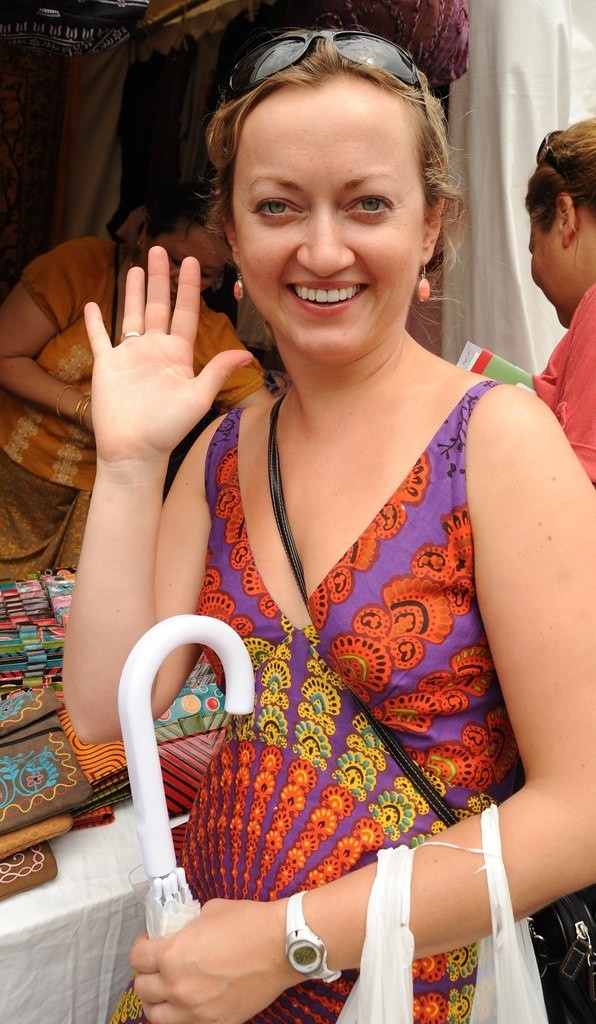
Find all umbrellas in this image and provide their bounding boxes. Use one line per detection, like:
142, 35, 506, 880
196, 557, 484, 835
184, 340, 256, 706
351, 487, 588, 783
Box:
117, 613, 255, 944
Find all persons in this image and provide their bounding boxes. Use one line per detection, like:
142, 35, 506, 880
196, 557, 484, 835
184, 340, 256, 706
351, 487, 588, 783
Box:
0, 180, 273, 582
525, 118, 596, 486
63, 29, 596, 1024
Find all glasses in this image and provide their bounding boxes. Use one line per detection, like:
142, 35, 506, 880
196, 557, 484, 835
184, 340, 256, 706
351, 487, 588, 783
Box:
537, 128, 578, 208
223, 30, 428, 123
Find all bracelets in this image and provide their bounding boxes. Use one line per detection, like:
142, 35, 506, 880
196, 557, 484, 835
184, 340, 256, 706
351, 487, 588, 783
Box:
74, 395, 91, 426
56, 385, 72, 418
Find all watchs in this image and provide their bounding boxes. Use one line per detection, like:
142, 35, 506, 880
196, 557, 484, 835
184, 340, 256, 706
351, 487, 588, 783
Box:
285, 891, 342, 983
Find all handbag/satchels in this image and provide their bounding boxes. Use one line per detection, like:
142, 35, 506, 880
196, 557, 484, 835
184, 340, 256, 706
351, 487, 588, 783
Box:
526, 892, 596, 1024
331, 802, 548, 1024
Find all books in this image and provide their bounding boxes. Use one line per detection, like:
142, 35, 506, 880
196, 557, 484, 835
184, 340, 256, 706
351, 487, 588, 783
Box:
456, 341, 536, 391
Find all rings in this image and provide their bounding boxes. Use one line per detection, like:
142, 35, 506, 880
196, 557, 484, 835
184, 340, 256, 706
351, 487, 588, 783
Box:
121, 331, 143, 341
